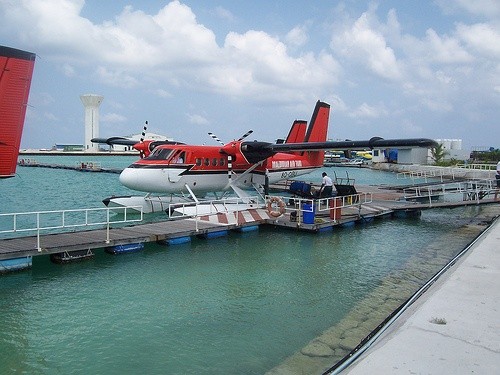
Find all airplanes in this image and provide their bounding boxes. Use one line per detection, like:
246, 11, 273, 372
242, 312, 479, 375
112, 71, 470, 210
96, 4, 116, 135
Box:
90, 100, 441, 218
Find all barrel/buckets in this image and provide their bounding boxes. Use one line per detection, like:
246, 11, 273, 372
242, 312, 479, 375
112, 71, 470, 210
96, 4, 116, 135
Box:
290, 212, 296, 221
303, 204, 315, 223
290, 182, 311, 193
330, 199, 341, 219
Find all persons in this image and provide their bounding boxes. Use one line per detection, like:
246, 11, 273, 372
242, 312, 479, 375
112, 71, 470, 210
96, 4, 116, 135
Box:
320, 172, 338, 205
493, 160, 500, 200
169, 152, 183, 163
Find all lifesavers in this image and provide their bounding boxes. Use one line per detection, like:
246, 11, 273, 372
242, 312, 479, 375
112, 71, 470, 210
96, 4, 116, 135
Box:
267, 197, 286, 217
